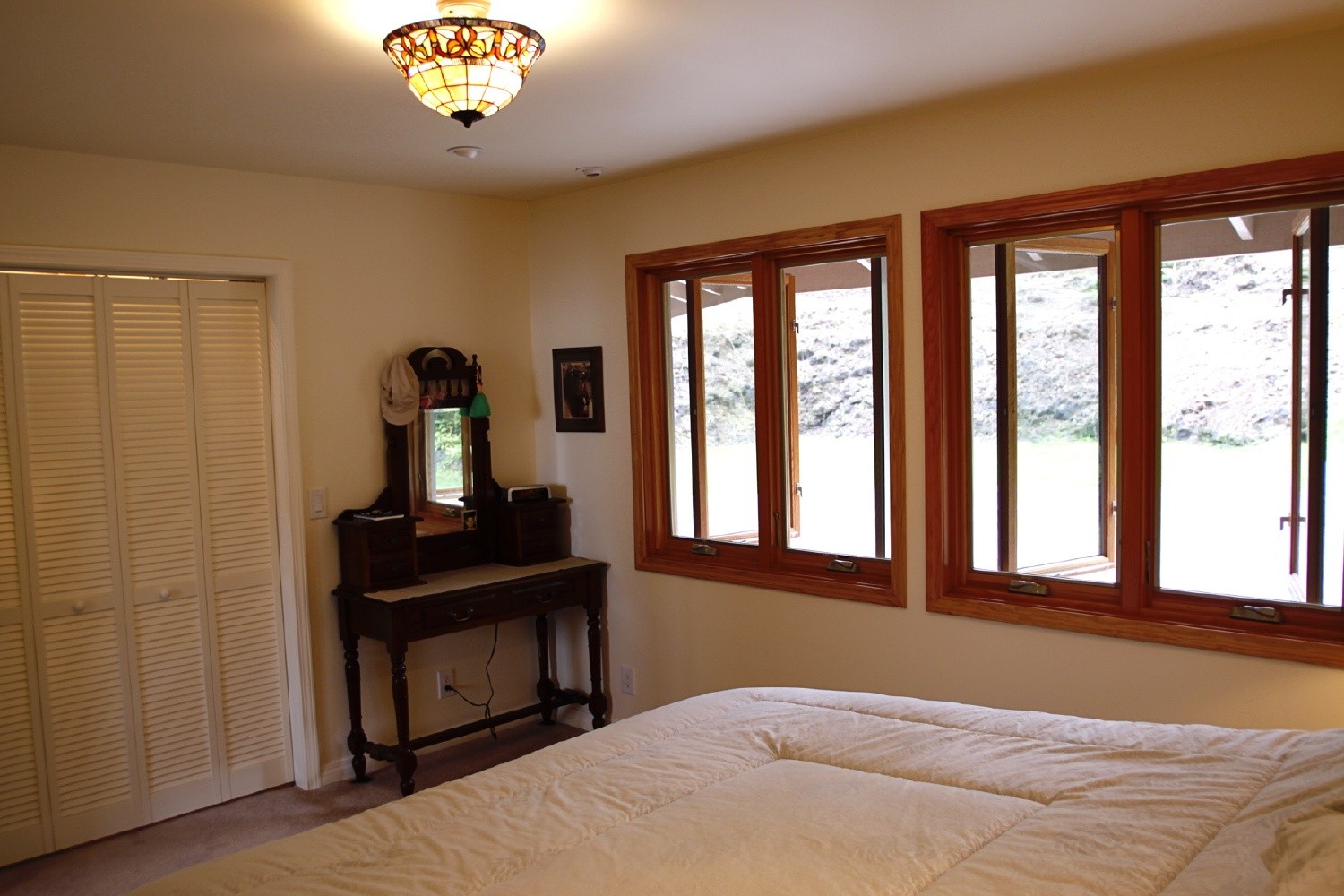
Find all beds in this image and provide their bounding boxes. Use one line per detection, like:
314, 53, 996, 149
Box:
121, 685, 1342, 896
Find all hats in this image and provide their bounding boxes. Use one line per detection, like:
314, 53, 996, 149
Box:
377, 353, 421, 425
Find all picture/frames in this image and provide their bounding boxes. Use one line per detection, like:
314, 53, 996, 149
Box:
552, 345, 605, 432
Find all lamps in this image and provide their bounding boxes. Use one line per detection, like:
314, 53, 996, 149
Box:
468, 366, 491, 418
383, 0, 546, 129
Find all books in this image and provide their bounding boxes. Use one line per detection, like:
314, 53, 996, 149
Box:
353, 512, 405, 521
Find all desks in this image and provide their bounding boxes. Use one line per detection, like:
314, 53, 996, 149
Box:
337, 558, 612, 801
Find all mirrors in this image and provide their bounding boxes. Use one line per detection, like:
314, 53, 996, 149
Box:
381, 345, 493, 541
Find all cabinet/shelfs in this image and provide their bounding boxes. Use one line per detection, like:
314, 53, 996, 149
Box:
333, 510, 423, 593
485, 489, 566, 566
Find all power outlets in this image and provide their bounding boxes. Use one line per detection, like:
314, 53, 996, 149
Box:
436, 669, 455, 699
621, 666, 635, 695
